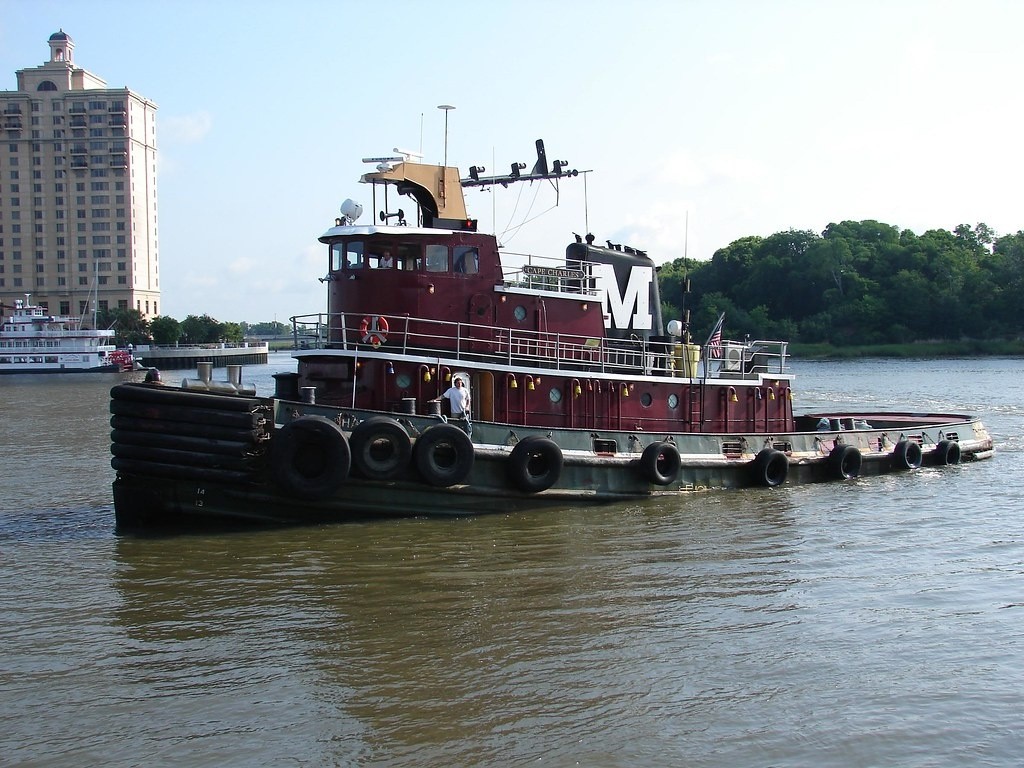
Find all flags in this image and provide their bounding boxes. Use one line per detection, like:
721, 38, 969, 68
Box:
702, 319, 723, 359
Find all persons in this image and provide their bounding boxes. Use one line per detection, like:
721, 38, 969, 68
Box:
427, 378, 470, 418
377, 250, 393, 267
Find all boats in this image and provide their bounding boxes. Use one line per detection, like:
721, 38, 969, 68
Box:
1, 258, 157, 375
102, 349, 134, 373
108, 109, 995, 541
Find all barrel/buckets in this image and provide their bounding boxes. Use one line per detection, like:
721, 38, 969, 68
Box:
677, 344, 700, 377
647, 334, 676, 377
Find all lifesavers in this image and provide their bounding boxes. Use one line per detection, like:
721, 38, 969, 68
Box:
754, 447, 790, 488
411, 421, 475, 488
893, 441, 923, 470
641, 442, 682, 484
935, 440, 962, 466
505, 435, 564, 495
360, 315, 390, 347
272, 414, 352, 496
344, 415, 413, 481
829, 443, 863, 481
